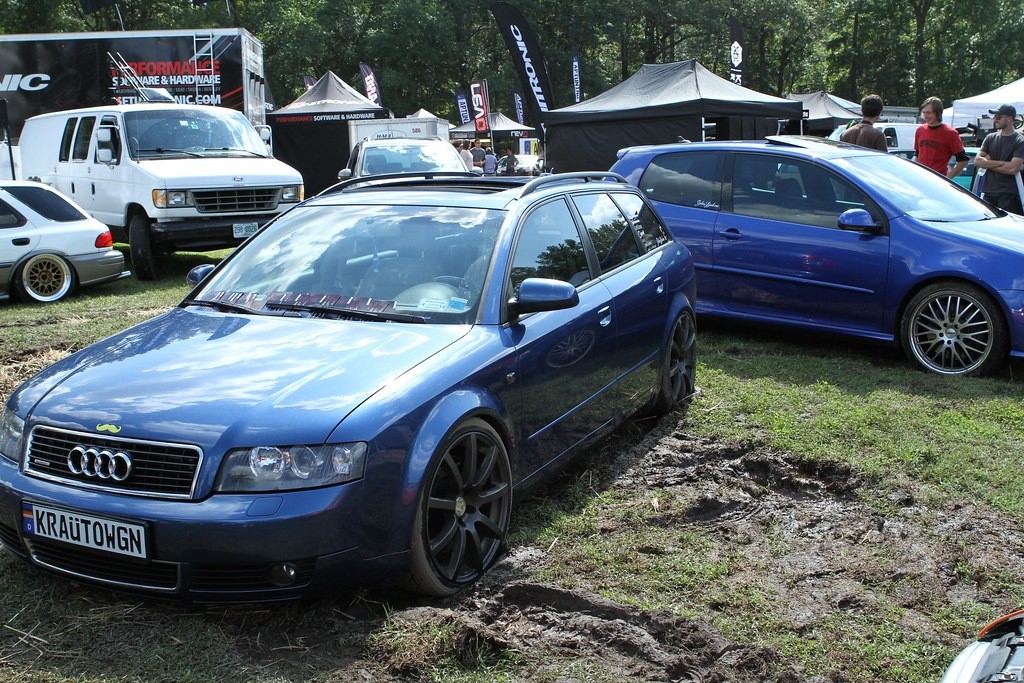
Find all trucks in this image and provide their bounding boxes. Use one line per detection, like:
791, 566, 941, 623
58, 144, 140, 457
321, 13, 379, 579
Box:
348, 118, 450, 164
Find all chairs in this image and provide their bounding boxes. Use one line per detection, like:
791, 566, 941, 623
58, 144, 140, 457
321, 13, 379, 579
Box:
368, 155, 389, 174
354, 216, 444, 302
773, 178, 814, 222
410, 162, 435, 169
461, 216, 550, 314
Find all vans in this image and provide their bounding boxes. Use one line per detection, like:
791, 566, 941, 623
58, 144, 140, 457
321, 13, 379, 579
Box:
825, 123, 923, 150
17, 103, 304, 282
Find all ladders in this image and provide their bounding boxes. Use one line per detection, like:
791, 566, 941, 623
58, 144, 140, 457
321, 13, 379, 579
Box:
193, 31, 215, 106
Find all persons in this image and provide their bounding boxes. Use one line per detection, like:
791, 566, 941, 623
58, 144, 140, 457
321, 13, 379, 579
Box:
973, 103, 1024, 215
910, 96, 971, 180
501, 146, 519, 175
459, 136, 499, 175
840, 93, 888, 153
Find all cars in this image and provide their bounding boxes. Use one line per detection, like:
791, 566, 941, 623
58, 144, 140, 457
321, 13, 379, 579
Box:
0, 180, 132, 303
775, 163, 840, 198
602, 134, 1023, 379
497, 155, 543, 173
887, 147, 981, 193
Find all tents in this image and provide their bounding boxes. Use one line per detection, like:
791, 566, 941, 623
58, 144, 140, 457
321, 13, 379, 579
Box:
942, 77, 1024, 147
265, 69, 390, 201
540, 56, 889, 174
405, 107, 536, 176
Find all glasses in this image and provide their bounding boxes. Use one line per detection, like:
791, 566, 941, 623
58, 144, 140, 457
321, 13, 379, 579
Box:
993, 115, 1009, 120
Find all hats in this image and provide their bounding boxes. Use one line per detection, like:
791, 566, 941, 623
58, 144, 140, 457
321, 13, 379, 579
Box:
988, 105, 1016, 117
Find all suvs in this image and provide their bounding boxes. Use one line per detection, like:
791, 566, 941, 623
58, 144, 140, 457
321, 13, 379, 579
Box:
0, 171, 698, 608
337, 137, 484, 188
